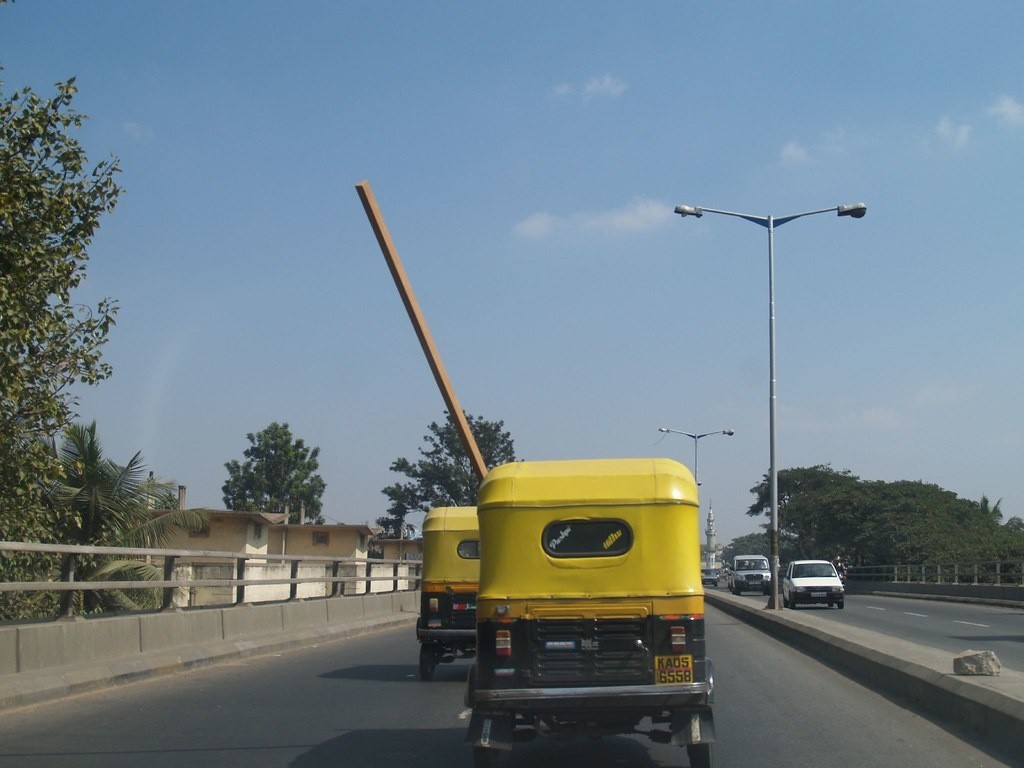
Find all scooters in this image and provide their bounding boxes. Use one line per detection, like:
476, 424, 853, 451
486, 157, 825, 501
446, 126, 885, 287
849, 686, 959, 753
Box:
831, 561, 848, 590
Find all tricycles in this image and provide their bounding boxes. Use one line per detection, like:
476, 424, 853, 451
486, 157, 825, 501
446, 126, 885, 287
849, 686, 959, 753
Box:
415, 506, 479, 676
467, 458, 718, 768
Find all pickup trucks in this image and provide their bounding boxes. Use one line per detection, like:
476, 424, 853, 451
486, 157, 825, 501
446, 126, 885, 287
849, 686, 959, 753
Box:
728, 554, 772, 595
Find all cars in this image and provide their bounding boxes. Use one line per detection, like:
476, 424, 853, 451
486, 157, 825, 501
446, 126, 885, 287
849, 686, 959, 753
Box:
782, 560, 845, 610
701, 568, 720, 587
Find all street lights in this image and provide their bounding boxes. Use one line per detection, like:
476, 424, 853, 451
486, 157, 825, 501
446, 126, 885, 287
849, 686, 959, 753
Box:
659, 427, 735, 486
673, 201, 867, 611
